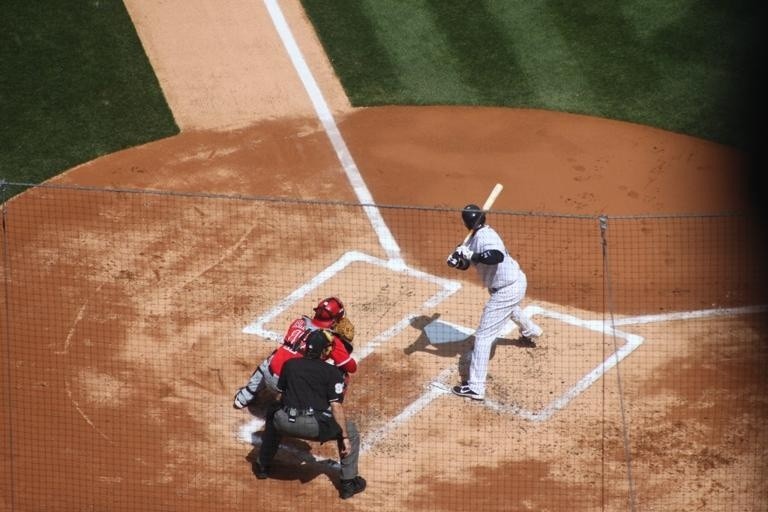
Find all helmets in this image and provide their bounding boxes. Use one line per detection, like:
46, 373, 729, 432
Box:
313, 298, 345, 326
461, 204, 485, 228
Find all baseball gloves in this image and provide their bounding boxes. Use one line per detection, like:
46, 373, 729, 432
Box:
332, 318, 353, 341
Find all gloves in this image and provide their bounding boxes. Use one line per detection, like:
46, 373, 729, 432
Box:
446, 245, 472, 267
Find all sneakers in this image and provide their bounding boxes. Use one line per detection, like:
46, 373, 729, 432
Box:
234, 388, 247, 410
523, 327, 545, 337
452, 384, 485, 400
343, 475, 366, 496
251, 451, 272, 478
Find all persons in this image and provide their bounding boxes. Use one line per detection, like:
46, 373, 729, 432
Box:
234, 297, 357, 409
253, 329, 367, 500
447, 205, 543, 400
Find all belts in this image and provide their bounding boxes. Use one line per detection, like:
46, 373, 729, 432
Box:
282, 406, 305, 416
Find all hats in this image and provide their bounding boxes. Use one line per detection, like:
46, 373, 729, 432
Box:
303, 328, 334, 360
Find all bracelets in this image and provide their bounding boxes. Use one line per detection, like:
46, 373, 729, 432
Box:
342, 436, 350, 439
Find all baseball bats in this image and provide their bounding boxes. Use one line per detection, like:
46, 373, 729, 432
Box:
447, 184, 502, 266
